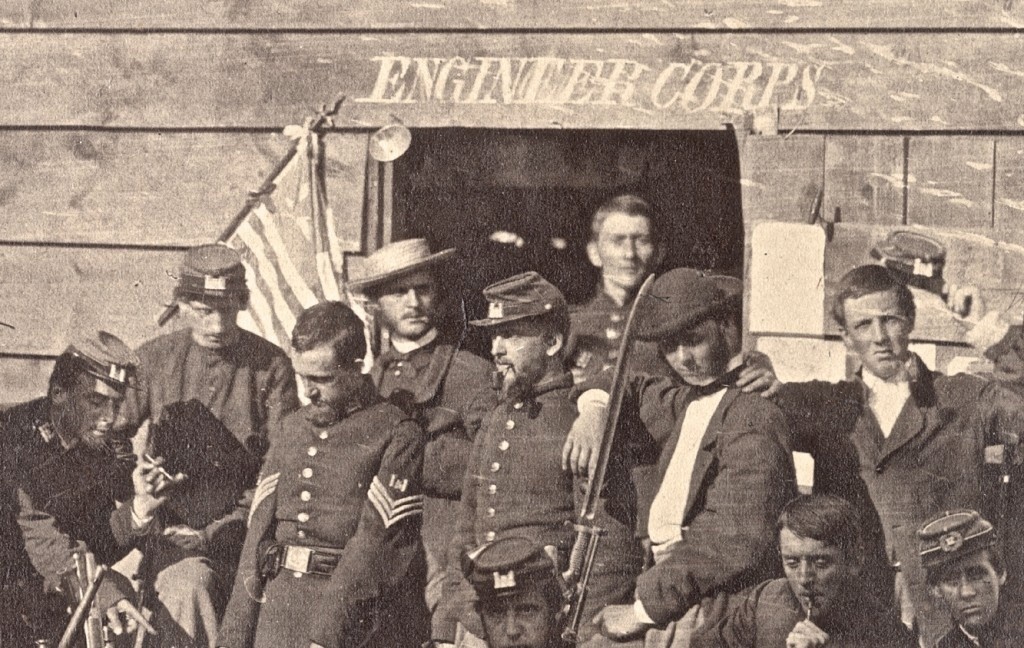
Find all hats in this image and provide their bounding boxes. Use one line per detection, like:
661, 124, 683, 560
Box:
176, 243, 249, 298
870, 231, 952, 299
346, 237, 457, 291
468, 272, 567, 325
916, 509, 1000, 568
469, 535, 556, 601
67, 331, 141, 391
634, 268, 745, 341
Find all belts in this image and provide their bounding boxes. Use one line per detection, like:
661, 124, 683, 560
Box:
280, 545, 338, 575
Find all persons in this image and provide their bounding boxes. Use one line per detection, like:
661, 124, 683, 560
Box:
0, 192, 1024, 648
